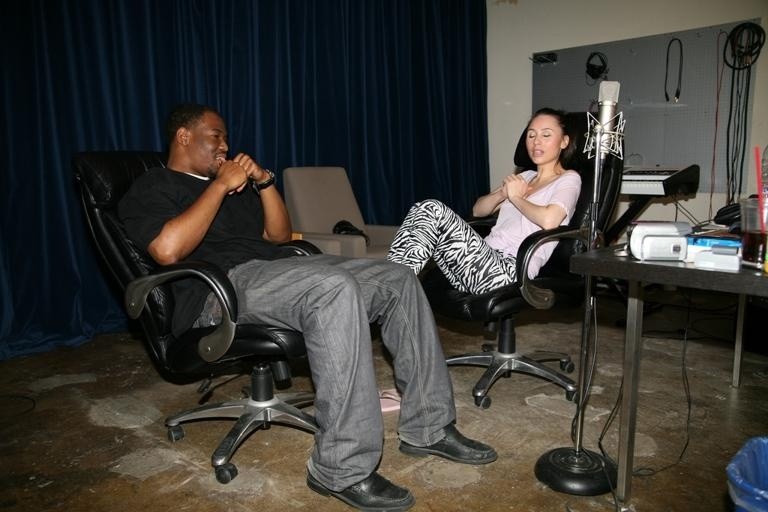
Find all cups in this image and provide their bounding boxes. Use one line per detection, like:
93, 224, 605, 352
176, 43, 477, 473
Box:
740, 198, 767, 270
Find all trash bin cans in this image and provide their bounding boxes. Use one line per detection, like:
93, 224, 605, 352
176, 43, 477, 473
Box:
725, 436, 768, 512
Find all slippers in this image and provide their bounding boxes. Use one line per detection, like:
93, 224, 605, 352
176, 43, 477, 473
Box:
377, 389, 401, 412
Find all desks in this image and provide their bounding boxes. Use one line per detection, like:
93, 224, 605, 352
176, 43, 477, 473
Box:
568, 243, 768, 512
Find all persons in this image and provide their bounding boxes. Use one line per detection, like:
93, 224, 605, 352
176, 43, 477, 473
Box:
118, 103, 497, 511
387, 108, 583, 296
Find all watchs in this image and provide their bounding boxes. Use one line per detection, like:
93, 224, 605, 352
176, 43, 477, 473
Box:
253, 168, 275, 191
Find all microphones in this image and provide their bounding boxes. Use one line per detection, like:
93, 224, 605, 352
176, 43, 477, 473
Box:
583, 80, 627, 160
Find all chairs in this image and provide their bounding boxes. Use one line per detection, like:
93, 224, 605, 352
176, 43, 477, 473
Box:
283, 167, 399, 259
421, 112, 624, 410
70, 151, 380, 484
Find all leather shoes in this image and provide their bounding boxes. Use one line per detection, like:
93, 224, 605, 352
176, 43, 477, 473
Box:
306, 470, 415, 512
399, 424, 497, 465
